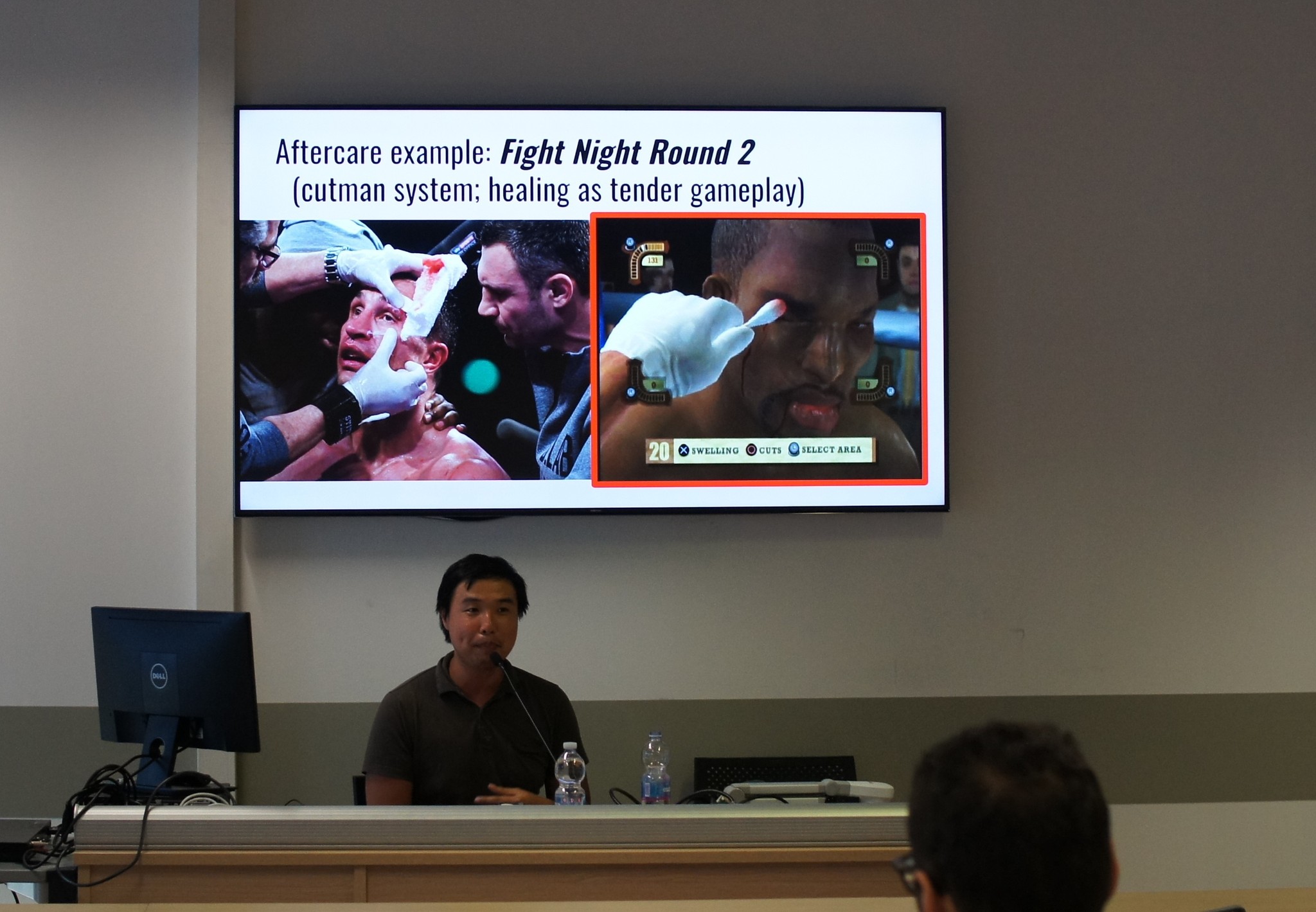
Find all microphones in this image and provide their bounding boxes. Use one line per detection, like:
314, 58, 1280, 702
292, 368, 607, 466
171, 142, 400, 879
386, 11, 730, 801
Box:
490, 652, 557, 762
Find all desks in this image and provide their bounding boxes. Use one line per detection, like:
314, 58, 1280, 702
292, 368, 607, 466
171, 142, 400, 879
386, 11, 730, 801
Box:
73, 802, 912, 905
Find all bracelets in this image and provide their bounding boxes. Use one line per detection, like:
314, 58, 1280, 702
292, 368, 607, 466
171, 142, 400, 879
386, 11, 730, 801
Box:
324, 245, 356, 288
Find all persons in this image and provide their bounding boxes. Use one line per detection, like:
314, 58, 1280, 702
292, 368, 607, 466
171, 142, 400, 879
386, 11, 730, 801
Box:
895, 718, 1120, 912
598, 287, 756, 448
477, 220, 593, 479
423, 391, 468, 432
876, 233, 922, 412
264, 275, 513, 482
362, 553, 591, 806
595, 218, 920, 480
238, 220, 429, 482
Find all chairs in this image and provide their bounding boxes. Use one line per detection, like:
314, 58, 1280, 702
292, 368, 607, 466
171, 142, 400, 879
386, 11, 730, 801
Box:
694, 755, 858, 804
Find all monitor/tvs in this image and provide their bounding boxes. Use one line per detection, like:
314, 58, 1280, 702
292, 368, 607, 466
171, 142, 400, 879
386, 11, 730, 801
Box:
232, 103, 952, 519
89, 605, 261, 799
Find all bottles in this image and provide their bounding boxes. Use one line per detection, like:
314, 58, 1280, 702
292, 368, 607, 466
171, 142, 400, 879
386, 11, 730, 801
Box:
554, 741, 586, 805
641, 731, 672, 804
